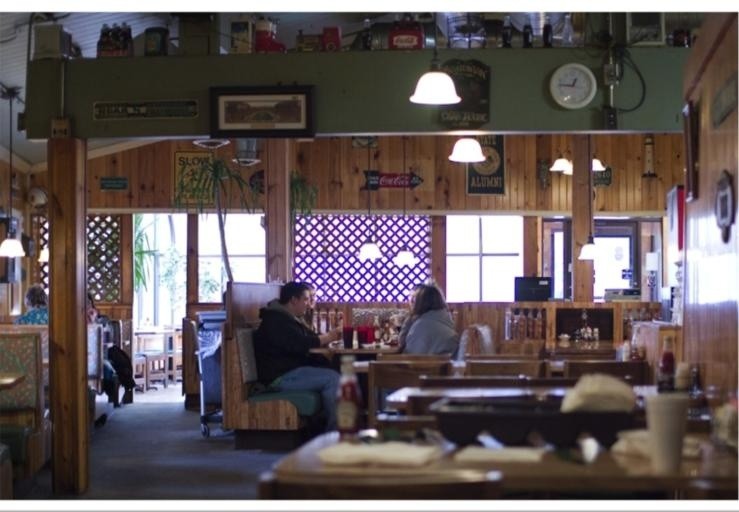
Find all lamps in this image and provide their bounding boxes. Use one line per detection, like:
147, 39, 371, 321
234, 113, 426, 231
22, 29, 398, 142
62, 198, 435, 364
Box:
546, 135, 572, 173
411, 12, 464, 106
579, 134, 601, 262
394, 133, 416, 272
0, 85, 25, 261
356, 134, 382, 261
444, 133, 487, 165
591, 133, 607, 176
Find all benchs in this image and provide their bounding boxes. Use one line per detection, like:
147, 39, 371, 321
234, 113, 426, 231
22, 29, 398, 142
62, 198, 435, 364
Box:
0, 315, 184, 499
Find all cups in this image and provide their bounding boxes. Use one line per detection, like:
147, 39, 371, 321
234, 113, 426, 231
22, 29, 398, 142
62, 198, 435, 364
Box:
642, 392, 691, 474
561, 340, 571, 347
559, 336, 571, 339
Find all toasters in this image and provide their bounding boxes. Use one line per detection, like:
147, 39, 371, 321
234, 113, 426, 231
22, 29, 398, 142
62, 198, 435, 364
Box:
373, 315, 382, 343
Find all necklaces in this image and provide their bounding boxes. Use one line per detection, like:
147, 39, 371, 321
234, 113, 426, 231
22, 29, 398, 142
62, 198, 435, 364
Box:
14, 285, 107, 431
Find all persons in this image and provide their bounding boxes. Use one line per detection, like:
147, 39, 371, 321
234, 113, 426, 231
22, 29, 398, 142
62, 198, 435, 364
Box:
252, 280, 341, 433
86, 291, 136, 408
402, 286, 458, 359
297, 282, 330, 347
398, 284, 424, 352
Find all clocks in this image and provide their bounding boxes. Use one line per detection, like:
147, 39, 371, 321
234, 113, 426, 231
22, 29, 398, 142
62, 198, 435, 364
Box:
549, 61, 597, 110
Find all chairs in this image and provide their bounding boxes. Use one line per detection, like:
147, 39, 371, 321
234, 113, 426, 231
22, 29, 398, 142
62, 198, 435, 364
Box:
180, 277, 738, 499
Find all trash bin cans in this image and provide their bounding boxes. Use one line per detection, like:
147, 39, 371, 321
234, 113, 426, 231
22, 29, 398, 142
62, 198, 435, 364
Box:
194, 311, 226, 436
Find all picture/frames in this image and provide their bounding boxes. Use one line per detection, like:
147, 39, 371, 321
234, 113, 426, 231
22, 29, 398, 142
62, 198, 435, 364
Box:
680, 101, 699, 203
460, 133, 507, 198
208, 81, 316, 141
708, 70, 738, 128
622, 12, 668, 48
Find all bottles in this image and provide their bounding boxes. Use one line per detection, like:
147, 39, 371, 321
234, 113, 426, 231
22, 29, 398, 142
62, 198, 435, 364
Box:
657, 333, 675, 393
353, 331, 359, 351
621, 339, 646, 362
573, 326, 601, 341
338, 355, 361, 439
